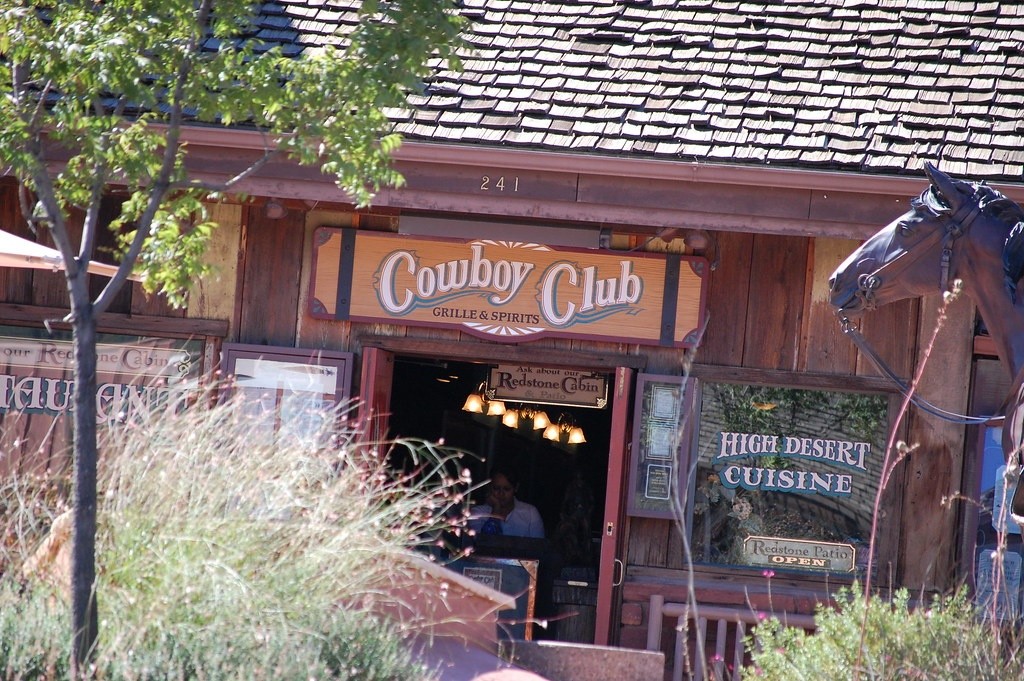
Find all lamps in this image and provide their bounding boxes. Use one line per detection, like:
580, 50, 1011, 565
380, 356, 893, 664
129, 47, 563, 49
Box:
436, 368, 450, 383
450, 366, 458, 379
461, 365, 507, 415
502, 402, 550, 430
543, 409, 586, 444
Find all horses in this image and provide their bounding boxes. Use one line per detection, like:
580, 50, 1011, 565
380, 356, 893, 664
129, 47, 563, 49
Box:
827, 160, 1024, 544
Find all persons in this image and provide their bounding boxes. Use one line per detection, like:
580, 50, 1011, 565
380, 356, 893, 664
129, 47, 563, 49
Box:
470, 468, 545, 538
